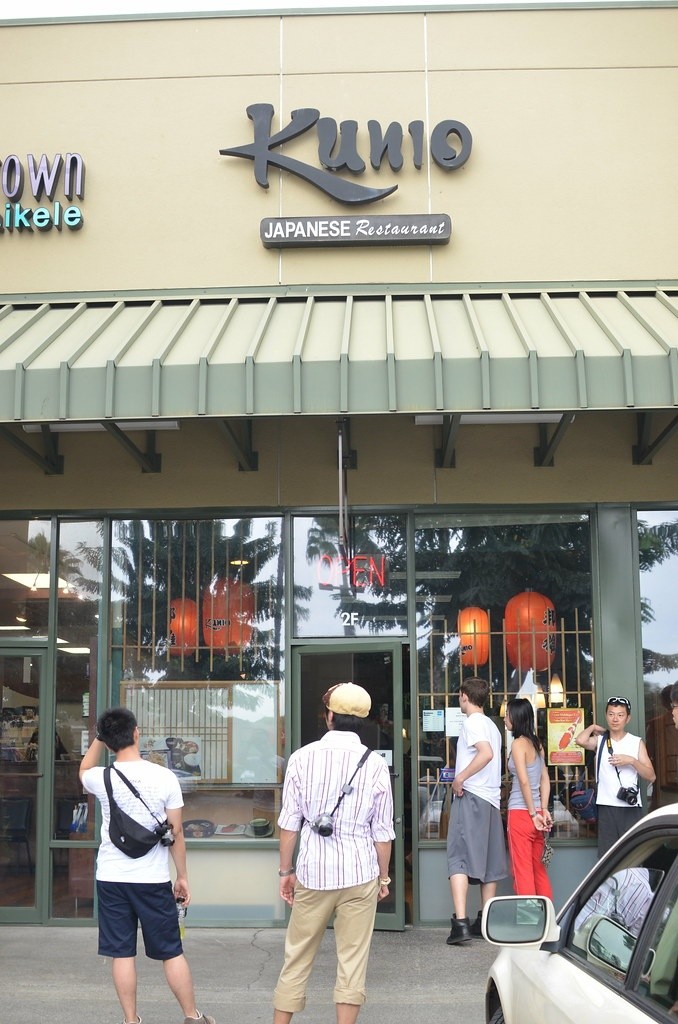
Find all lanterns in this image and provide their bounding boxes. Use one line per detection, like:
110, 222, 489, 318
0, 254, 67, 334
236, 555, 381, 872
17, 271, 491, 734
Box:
457, 605, 490, 667
203, 574, 254, 658
505, 588, 555, 676
170, 597, 196, 656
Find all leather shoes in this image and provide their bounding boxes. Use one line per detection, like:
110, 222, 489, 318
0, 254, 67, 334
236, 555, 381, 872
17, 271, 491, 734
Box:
471, 910, 484, 936
446, 913, 471, 943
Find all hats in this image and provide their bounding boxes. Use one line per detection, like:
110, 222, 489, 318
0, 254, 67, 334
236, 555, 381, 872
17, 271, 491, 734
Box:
322, 683, 372, 719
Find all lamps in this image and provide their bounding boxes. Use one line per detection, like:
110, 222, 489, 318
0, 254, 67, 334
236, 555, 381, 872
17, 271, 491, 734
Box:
63, 582, 69, 593
30, 571, 39, 591
229, 542, 249, 565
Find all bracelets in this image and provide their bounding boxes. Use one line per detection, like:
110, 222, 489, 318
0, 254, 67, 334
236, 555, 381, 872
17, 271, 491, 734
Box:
542, 808, 548, 809
381, 877, 391, 885
530, 811, 538, 818
278, 866, 294, 876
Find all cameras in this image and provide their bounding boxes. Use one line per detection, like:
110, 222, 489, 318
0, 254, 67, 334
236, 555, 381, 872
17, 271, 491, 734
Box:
309, 812, 336, 837
617, 787, 638, 805
155, 824, 175, 847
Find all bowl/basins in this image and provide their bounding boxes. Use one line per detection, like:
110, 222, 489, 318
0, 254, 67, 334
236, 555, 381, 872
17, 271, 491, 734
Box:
166, 738, 200, 770
182, 819, 213, 839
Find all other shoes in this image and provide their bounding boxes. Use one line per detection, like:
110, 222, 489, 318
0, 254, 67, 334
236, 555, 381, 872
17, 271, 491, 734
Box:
184, 1009, 215, 1024
123, 1014, 141, 1024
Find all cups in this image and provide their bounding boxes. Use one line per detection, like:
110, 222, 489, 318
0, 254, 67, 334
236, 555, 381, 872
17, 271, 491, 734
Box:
249, 818, 270, 835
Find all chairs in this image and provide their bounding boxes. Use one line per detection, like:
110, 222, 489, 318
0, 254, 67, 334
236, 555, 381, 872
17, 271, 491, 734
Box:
0, 799, 33, 878
55, 798, 88, 870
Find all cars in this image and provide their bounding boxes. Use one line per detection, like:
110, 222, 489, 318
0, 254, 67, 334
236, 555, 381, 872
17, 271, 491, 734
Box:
480, 801, 678, 1024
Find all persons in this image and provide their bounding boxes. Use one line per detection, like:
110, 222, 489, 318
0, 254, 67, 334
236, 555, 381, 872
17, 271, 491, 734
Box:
79, 707, 214, 1024
670, 681, 678, 730
503, 699, 556, 903
446, 677, 507, 943
573, 868, 654, 971
576, 697, 655, 860
273, 682, 396, 1024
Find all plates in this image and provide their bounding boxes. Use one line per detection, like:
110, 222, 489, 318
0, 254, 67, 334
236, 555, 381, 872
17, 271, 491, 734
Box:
213, 824, 245, 836
245, 825, 274, 837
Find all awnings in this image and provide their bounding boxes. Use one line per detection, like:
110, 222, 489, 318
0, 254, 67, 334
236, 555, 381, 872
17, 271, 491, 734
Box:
0, 293, 678, 471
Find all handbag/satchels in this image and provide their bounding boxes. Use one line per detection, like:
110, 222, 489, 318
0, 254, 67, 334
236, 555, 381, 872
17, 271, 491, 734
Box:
108, 800, 159, 859
540, 832, 554, 868
570, 789, 601, 825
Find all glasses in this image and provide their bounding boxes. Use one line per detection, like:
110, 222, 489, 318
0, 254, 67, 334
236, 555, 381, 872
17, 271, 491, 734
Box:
671, 703, 678, 709
607, 694, 630, 710
